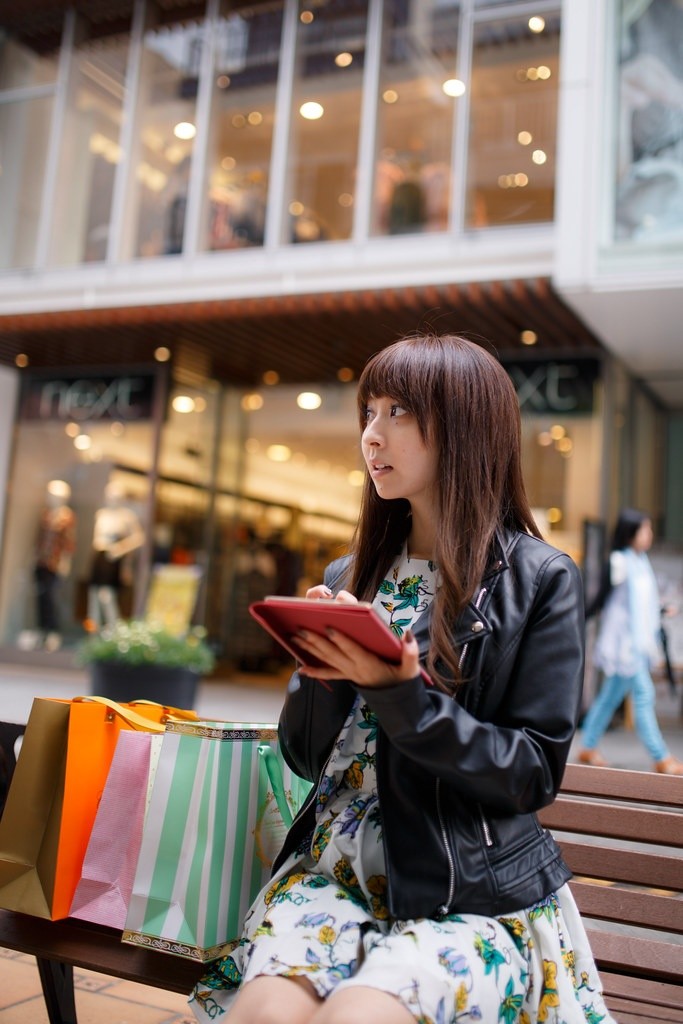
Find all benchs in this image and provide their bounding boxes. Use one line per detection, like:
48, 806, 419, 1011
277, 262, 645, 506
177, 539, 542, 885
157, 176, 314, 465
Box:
0, 708, 683, 1024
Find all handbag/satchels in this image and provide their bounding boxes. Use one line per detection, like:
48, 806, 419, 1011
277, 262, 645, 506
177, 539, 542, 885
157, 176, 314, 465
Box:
0, 693, 314, 965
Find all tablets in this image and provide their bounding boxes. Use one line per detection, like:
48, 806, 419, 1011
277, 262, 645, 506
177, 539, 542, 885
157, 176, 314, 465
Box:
266, 596, 436, 689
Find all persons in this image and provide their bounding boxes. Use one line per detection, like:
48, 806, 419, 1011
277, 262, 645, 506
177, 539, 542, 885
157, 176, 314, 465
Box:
188, 334, 617, 1024
21, 473, 148, 653
579, 508, 683, 775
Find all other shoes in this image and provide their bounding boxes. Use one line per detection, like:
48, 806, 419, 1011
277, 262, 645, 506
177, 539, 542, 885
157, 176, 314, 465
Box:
654, 756, 683, 776
572, 748, 607, 767
